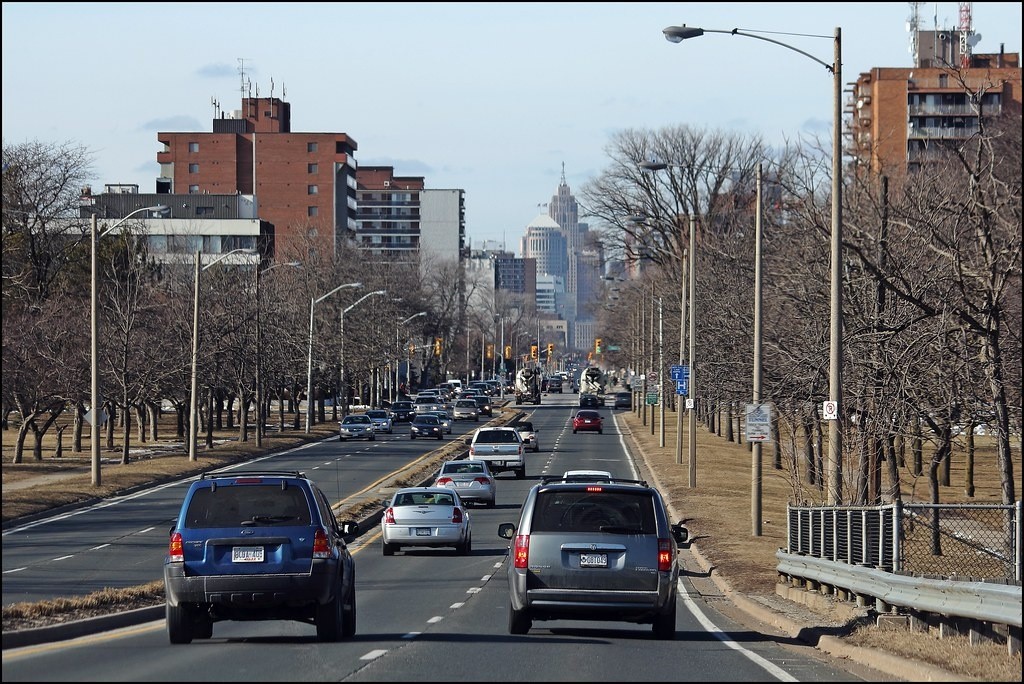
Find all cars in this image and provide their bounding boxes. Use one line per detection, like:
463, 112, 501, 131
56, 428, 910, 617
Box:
465, 427, 531, 480
337, 372, 632, 442
382, 486, 472, 556
433, 460, 498, 508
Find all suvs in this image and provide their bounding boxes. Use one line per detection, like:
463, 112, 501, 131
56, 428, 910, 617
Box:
497, 476, 689, 637
164, 469, 358, 644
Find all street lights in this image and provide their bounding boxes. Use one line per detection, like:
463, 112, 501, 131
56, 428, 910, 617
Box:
90, 204, 173, 488
255, 263, 428, 447
600, 276, 646, 425
638, 162, 763, 536
630, 244, 688, 461
663, 27, 841, 505
625, 216, 698, 490
188, 248, 258, 460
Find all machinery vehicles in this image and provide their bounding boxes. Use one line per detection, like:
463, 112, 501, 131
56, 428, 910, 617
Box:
580, 368, 605, 405
514, 368, 542, 405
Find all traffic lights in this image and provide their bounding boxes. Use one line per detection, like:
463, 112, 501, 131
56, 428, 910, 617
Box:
595, 339, 603, 355
531, 346, 538, 358
548, 344, 553, 356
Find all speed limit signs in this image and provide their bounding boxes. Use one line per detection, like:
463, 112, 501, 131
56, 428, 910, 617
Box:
635, 379, 643, 385
